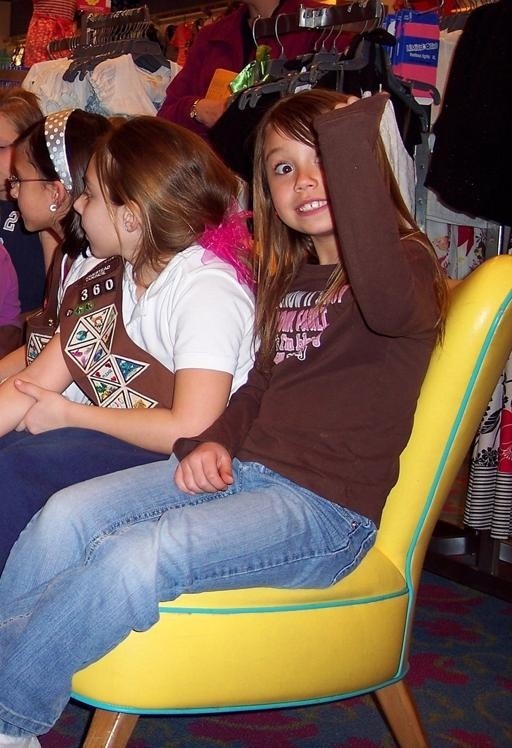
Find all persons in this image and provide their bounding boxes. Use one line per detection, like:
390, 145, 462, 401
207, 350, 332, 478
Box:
1, 115, 261, 583
0, 86, 64, 361
0, 107, 113, 385
1, 87, 449, 743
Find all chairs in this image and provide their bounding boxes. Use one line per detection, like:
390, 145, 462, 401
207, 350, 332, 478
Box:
68, 254, 512, 748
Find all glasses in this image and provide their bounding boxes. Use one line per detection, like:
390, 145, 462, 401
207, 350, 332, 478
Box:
7, 175, 59, 189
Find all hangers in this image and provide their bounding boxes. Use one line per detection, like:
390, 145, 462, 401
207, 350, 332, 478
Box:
237, 2, 441, 109
46, 8, 170, 83
